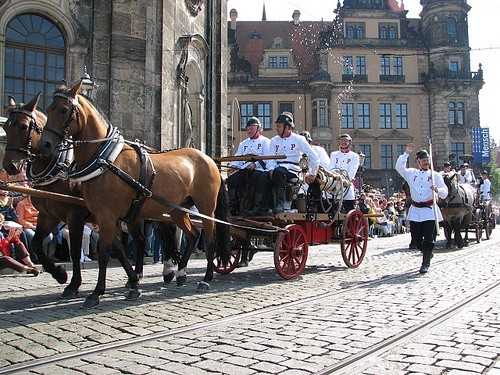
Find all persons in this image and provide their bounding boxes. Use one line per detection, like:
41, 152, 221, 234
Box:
297, 129, 330, 186
325, 129, 358, 209
356, 181, 408, 239
272, 111, 320, 213
226, 115, 273, 213
438, 160, 490, 209
0, 174, 102, 271
393, 142, 449, 275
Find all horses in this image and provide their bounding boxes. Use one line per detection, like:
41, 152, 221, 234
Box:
402, 173, 475, 250
2, 78, 232, 310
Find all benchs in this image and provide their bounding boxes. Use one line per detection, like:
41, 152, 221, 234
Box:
286, 168, 303, 213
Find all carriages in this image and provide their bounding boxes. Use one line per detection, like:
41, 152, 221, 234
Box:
403, 173, 496, 250
0, 77, 385, 310
